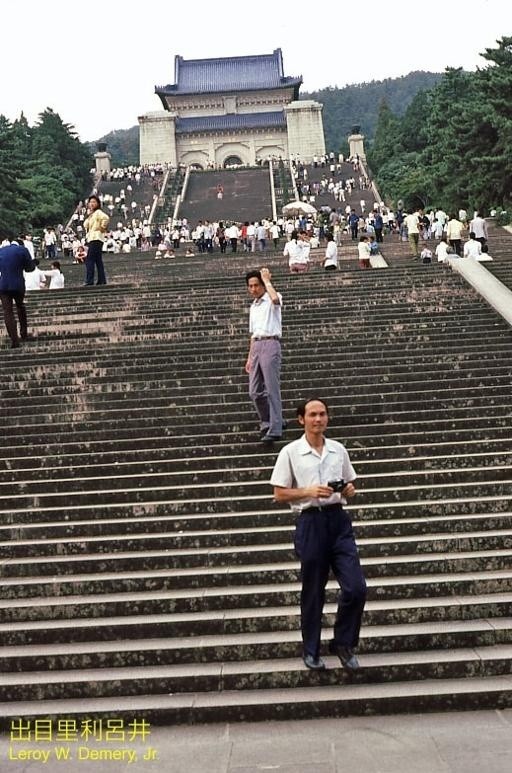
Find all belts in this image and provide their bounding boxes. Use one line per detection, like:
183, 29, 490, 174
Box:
253, 334, 280, 341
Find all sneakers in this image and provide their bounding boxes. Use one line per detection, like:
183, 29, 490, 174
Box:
80, 280, 107, 287
11, 333, 28, 349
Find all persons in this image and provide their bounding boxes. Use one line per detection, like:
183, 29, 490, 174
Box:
195, 154, 280, 171
500, 208, 507, 215
267, 397, 368, 674
244, 265, 285, 443
216, 183, 224, 198
1, 239, 40, 348
59, 159, 188, 263
490, 206, 497, 216
78, 194, 108, 287
1, 224, 59, 289
281, 149, 372, 199
189, 198, 492, 272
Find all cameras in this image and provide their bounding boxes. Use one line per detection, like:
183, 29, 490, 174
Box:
327, 477, 345, 493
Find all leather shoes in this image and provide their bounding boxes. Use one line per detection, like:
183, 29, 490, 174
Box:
261, 433, 285, 442
332, 638, 362, 673
298, 648, 327, 672
